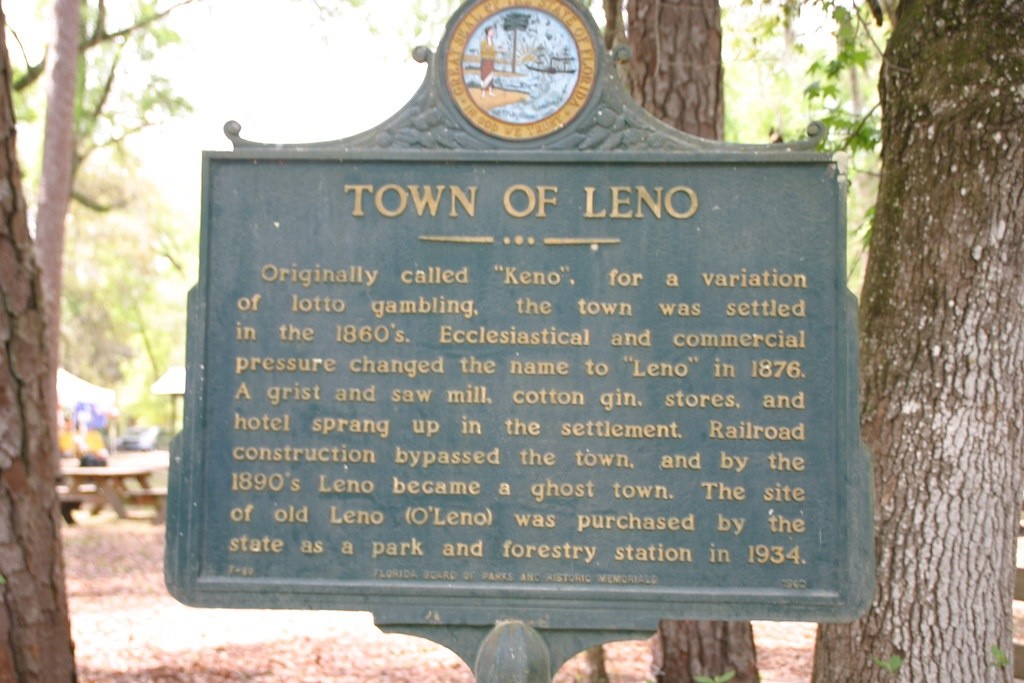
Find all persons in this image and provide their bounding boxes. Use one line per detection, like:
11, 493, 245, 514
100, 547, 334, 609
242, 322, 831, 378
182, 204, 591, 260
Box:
54, 407, 111, 526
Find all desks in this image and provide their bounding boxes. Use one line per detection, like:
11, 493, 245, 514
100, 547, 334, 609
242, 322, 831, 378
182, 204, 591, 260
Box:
62, 467, 166, 518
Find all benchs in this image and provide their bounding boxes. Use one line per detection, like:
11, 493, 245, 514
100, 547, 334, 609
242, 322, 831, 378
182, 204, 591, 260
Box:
60, 487, 169, 503
